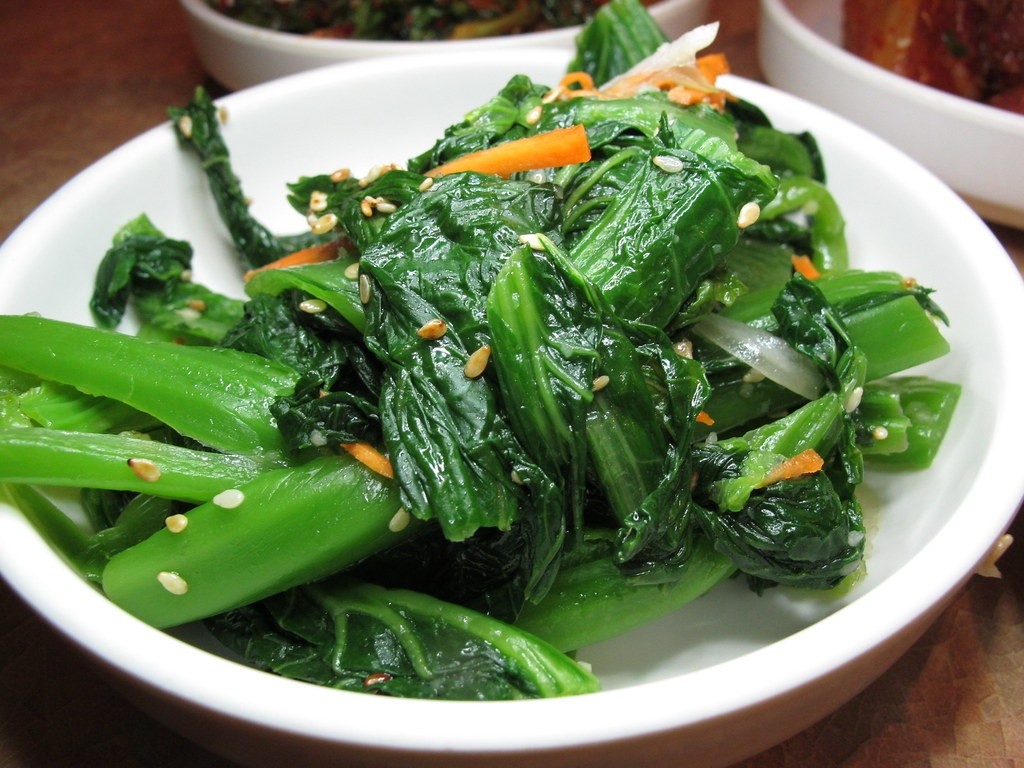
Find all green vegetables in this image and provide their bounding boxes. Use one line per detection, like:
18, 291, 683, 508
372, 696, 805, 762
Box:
1, 0, 961, 698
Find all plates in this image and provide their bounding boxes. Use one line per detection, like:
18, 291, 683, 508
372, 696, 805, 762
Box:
0, 53, 1024, 768
179, 0, 701, 89
759, 0, 1024, 228
